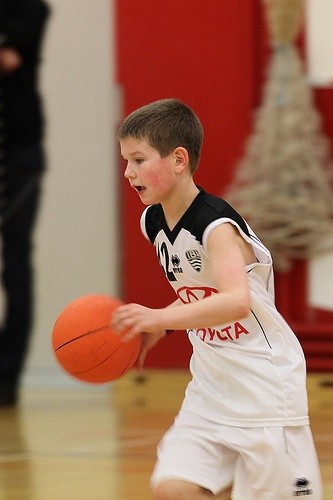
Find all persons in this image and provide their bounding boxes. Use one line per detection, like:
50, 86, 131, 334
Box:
0, 0, 54, 406
108, 97, 324, 500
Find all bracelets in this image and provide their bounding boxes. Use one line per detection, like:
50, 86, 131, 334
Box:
165, 328, 175, 334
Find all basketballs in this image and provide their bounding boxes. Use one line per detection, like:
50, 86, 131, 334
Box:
51, 295, 142, 385
